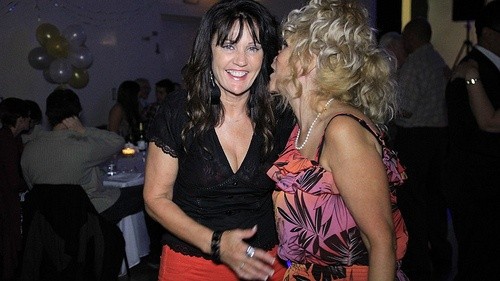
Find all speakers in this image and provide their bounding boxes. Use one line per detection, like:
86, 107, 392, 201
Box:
452, 0, 486, 22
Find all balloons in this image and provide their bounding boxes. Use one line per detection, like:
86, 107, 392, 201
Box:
28, 23, 93, 89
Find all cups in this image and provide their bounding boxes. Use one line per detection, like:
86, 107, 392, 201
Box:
107, 160, 116, 176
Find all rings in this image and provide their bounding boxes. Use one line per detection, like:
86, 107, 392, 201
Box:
241, 263, 244, 269
245, 246, 255, 258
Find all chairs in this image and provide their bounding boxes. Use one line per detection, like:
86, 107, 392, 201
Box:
29, 184, 133, 281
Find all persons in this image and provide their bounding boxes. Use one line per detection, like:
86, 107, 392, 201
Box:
0, 75, 177, 281
379, 1, 500, 281
265, 0, 409, 281
142, 0, 299, 281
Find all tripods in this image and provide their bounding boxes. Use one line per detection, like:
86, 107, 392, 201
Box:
452, 21, 473, 67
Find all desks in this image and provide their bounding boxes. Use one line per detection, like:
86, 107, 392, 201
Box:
99, 172, 151, 278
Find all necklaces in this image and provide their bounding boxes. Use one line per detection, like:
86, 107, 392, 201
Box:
295, 96, 334, 149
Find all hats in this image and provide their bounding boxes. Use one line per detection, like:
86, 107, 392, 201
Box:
46, 88, 82, 125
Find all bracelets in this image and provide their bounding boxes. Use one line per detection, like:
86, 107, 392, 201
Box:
465, 78, 481, 85
211, 230, 224, 260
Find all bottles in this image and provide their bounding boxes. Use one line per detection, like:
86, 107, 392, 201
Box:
137, 122, 146, 151
